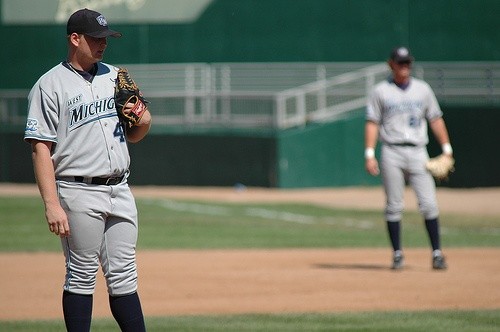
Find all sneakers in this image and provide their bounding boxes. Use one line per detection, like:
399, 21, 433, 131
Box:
393, 253, 404, 269
434, 255, 446, 270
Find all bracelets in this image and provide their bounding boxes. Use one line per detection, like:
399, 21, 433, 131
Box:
365, 148, 375, 158
441, 143, 453, 155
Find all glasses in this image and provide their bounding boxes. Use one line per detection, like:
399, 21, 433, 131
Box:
398, 59, 409, 64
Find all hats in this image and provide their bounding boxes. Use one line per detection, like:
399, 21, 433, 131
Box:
66, 7, 122, 40
391, 47, 412, 61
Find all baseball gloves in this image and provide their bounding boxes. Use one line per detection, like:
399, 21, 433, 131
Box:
114, 67, 147, 127
425, 154, 456, 181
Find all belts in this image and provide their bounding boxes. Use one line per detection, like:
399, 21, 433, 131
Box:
75, 176, 124, 186
395, 143, 415, 146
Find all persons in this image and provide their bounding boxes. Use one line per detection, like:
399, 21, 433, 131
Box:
24, 8, 152, 332
365, 46, 455, 270
